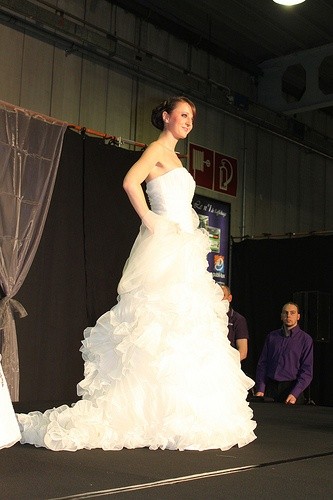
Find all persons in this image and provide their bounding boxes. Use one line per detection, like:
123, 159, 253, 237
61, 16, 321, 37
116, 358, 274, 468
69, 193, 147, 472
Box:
253, 301, 314, 404
15, 94, 257, 451
219, 284, 250, 377
